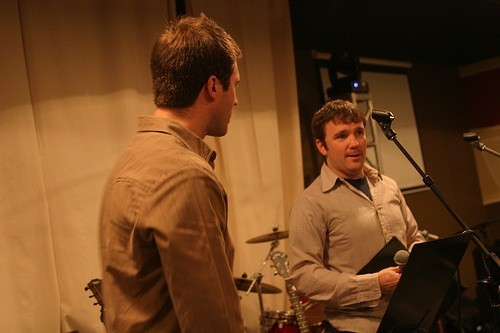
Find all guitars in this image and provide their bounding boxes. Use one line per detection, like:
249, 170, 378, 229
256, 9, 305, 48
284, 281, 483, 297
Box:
270, 251, 312, 333
85, 278, 103, 305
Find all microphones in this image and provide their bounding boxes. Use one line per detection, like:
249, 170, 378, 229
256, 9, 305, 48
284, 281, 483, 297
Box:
394, 250, 410, 270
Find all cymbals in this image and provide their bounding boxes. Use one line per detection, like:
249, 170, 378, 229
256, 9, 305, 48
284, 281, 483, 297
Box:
234, 278, 282, 294
245, 230, 289, 244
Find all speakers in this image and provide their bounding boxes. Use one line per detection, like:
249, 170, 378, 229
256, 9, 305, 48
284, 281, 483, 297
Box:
473, 243, 500, 317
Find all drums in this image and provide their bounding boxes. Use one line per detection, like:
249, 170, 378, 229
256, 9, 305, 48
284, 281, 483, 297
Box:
259, 310, 297, 333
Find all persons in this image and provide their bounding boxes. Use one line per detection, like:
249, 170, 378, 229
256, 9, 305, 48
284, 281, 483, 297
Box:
289, 100, 426, 333
98, 17, 250, 333
